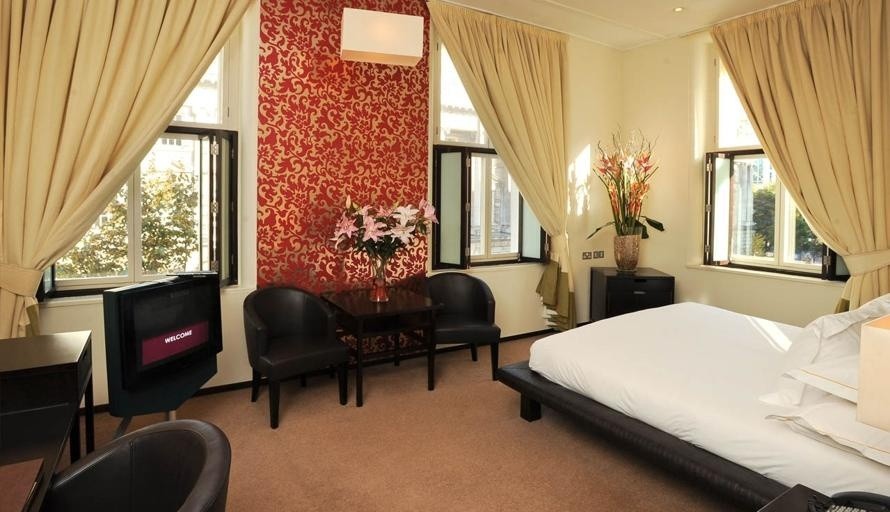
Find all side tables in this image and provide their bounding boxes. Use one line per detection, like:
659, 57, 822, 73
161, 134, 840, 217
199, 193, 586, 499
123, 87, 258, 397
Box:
319, 285, 445, 407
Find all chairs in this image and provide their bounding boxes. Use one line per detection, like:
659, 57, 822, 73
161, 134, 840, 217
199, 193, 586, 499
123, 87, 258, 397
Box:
416, 272, 500, 390
244, 287, 349, 429
38, 420, 231, 511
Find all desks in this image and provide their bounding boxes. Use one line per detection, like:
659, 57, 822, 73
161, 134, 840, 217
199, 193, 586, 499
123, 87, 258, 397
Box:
0, 330, 95, 512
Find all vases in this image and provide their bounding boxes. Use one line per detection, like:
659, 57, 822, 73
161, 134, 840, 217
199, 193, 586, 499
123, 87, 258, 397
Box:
369, 277, 389, 302
614, 235, 641, 274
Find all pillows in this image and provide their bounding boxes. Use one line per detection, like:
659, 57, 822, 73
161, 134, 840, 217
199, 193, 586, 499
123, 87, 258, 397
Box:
758, 293, 890, 467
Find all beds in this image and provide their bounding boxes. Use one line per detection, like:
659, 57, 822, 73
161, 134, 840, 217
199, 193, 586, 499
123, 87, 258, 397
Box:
498, 300, 889, 505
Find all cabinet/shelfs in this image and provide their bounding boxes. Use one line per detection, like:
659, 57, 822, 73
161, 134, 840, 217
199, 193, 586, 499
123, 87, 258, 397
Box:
590, 266, 674, 323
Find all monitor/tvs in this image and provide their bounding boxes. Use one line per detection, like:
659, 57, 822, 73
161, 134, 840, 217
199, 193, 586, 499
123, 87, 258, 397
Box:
120, 273, 223, 393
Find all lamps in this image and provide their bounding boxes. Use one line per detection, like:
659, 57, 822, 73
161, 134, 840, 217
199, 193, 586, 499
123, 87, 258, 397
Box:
340, 7, 424, 66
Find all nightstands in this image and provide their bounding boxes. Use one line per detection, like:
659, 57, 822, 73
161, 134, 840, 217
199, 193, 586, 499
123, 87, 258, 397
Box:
752, 484, 889, 512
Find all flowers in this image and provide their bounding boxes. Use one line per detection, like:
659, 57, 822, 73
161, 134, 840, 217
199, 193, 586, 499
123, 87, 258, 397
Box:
586, 120, 664, 239
328, 194, 439, 280
